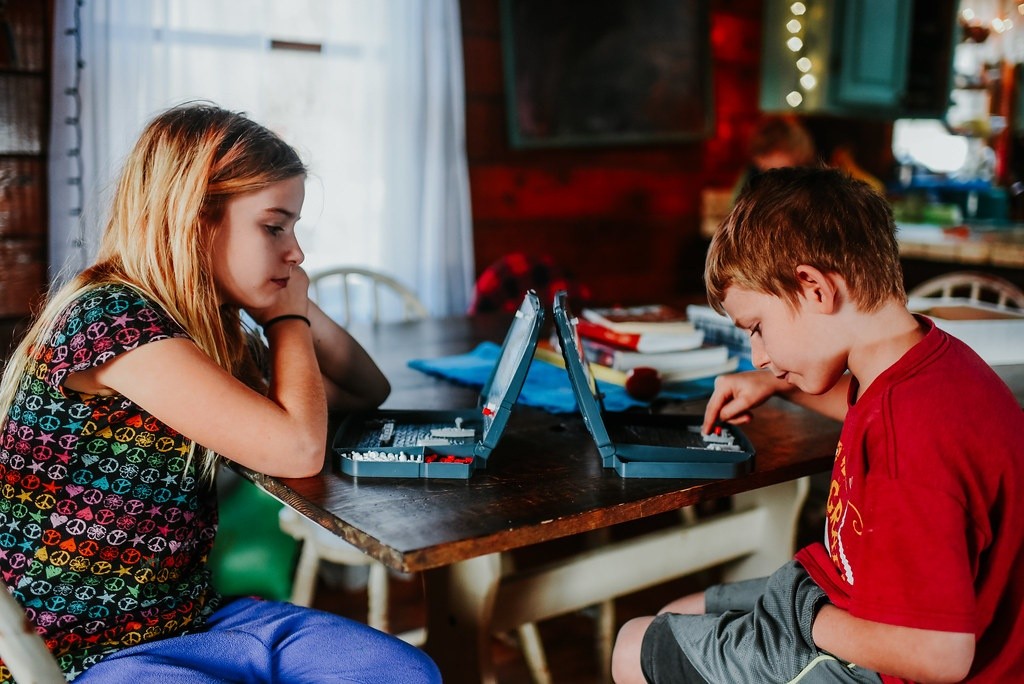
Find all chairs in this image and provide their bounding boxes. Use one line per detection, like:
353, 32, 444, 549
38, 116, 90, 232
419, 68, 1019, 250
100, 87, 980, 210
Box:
909, 275, 1024, 312
278, 265, 550, 684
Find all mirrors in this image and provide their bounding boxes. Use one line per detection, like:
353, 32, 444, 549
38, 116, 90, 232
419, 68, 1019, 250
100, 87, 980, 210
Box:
498, 0, 716, 150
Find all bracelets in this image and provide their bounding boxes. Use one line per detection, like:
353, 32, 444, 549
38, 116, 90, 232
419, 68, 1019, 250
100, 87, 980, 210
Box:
262, 315, 311, 337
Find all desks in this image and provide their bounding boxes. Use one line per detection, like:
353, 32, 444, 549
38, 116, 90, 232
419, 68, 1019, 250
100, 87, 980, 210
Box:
215, 296, 1024, 684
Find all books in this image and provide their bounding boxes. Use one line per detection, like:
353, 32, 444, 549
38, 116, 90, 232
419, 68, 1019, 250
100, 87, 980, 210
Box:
533, 304, 738, 388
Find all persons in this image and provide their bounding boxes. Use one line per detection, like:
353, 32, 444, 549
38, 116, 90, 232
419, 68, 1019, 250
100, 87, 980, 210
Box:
611, 165, 1024, 684
0, 105, 441, 684
